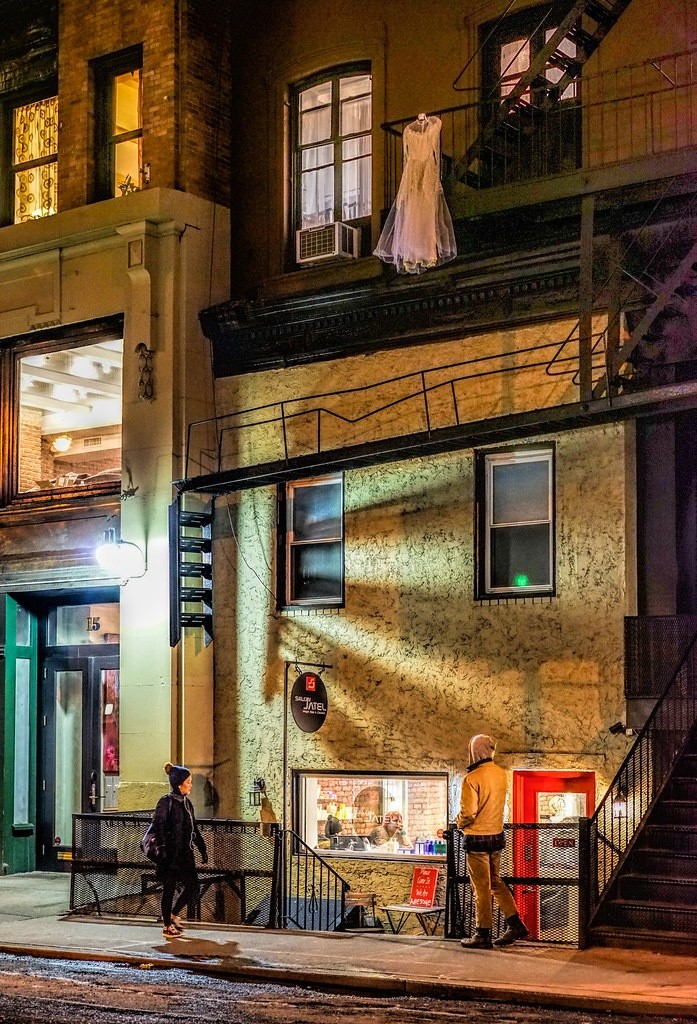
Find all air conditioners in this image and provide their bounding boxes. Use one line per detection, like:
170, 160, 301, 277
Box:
295, 222, 358, 267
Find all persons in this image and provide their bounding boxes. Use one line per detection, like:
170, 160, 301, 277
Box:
153, 764, 208, 938
371, 811, 414, 849
324, 815, 343, 837
456, 733, 529, 949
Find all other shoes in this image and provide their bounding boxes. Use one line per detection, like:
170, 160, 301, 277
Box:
460, 928, 494, 950
490, 914, 529, 945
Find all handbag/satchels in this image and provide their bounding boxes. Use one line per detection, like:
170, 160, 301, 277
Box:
140, 824, 164, 863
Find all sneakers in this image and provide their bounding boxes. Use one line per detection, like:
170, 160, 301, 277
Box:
170, 913, 182, 929
162, 925, 181, 938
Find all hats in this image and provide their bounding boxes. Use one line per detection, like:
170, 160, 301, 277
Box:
163, 762, 190, 788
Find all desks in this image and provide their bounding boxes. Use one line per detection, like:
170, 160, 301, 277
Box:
377, 904, 446, 937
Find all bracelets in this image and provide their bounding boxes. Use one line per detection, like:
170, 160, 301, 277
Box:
401, 831, 406, 834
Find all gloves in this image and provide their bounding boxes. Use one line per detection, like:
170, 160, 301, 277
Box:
156, 863, 168, 878
200, 853, 209, 866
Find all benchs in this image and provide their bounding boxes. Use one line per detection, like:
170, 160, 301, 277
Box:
344, 892, 388, 933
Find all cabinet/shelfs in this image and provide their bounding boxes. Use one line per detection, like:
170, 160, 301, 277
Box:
317, 797, 352, 843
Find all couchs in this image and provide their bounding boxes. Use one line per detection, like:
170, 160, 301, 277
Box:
540, 886, 569, 930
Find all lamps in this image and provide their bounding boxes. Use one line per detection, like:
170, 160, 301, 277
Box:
248, 777, 266, 806
94, 528, 144, 571
612, 785, 630, 820
133, 342, 155, 401
